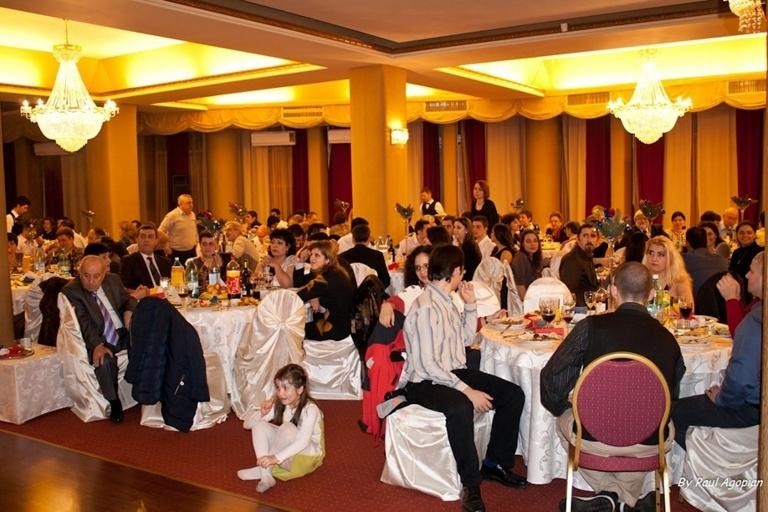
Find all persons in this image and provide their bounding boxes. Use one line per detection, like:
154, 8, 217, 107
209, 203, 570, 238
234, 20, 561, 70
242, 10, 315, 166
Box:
340, 226, 392, 303
645, 235, 694, 319
469, 179, 500, 224
242, 207, 350, 235
182, 233, 227, 290
538, 260, 686, 512
397, 219, 430, 259
471, 216, 495, 259
119, 226, 177, 298
561, 226, 607, 307
671, 251, 762, 449
378, 248, 464, 328
513, 231, 543, 301
662, 212, 689, 251
700, 212, 723, 235
488, 224, 516, 269
236, 364, 325, 492
156, 193, 198, 266
426, 225, 452, 247
500, 214, 524, 239
683, 226, 732, 295
297, 243, 357, 340
223, 222, 261, 282
721, 208, 738, 237
401, 246, 527, 512
731, 223, 765, 297
263, 230, 300, 287
452, 219, 483, 279
546, 212, 562, 242
699, 223, 730, 259
617, 210, 657, 261
517, 209, 540, 233
336, 217, 374, 254
5, 202, 136, 269
61, 255, 136, 422
568, 200, 615, 230
416, 188, 447, 219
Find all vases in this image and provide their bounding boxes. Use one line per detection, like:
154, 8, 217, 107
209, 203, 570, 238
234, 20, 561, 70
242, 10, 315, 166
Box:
404, 219, 409, 235
607, 238, 617, 268
741, 209, 744, 224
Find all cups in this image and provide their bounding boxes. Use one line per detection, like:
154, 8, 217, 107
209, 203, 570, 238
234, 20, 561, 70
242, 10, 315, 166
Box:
401, 248, 407, 261
562, 292, 577, 308
240, 290, 247, 299
678, 295, 694, 319
661, 290, 671, 308
553, 297, 561, 322
676, 320, 691, 337
252, 281, 261, 302
264, 272, 273, 290
175, 282, 190, 310
158, 276, 170, 297
583, 290, 596, 315
540, 306, 556, 329
538, 296, 554, 311
19, 337, 33, 348
15, 250, 24, 267
247, 277, 256, 297
562, 306, 575, 336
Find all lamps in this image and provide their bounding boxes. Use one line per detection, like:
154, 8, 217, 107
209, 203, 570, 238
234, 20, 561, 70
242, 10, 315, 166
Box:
21, 20, 118, 151
390, 127, 410, 144
606, 47, 692, 144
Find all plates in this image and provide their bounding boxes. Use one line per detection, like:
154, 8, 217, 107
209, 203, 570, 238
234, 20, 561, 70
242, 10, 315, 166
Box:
571, 312, 587, 323
709, 331, 731, 338
0, 343, 36, 360
490, 317, 532, 331
692, 314, 719, 323
518, 333, 558, 345
676, 336, 711, 348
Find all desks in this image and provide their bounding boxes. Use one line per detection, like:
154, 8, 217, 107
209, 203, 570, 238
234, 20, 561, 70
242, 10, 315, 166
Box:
0, 342, 74, 423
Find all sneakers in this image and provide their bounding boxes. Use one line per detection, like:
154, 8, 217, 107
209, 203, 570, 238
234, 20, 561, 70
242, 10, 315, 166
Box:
559, 496, 615, 512
615, 490, 656, 512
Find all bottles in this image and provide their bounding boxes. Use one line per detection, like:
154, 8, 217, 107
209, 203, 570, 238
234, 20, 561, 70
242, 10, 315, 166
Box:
71, 249, 79, 278
226, 255, 241, 298
171, 257, 186, 296
388, 239, 394, 263
646, 273, 659, 316
23, 252, 31, 276
50, 252, 59, 271
36, 243, 46, 272
209, 253, 220, 285
185, 258, 200, 298
241, 259, 250, 297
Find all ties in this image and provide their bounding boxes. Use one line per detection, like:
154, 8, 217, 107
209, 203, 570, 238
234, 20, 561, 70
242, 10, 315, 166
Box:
91, 291, 120, 347
147, 256, 160, 285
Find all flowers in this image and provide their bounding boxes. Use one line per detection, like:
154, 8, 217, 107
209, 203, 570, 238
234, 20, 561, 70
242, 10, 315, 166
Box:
730, 193, 758, 208
394, 202, 415, 218
228, 201, 249, 217
583, 205, 633, 238
639, 199, 665, 220
334, 199, 351, 209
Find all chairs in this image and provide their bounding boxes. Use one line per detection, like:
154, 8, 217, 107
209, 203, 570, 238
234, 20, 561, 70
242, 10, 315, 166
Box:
304, 275, 381, 401
56, 292, 138, 424
233, 290, 306, 425
377, 313, 493, 500
23, 272, 69, 347
130, 297, 231, 431
473, 257, 503, 300
525, 277, 573, 311
566, 351, 671, 512
504, 262, 528, 318
456, 280, 500, 317
680, 422, 759, 512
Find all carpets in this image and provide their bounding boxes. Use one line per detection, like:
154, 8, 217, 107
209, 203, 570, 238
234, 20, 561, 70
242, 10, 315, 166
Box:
0, 402, 699, 512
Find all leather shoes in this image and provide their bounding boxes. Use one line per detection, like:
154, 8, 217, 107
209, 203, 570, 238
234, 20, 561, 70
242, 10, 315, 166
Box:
480, 464, 528, 488
110, 400, 123, 423
461, 484, 485, 512
